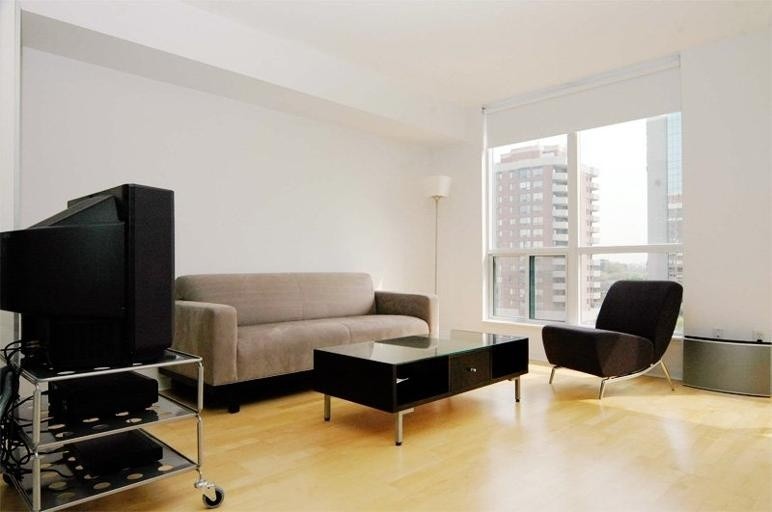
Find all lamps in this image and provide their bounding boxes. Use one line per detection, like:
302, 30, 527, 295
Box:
424, 175, 451, 295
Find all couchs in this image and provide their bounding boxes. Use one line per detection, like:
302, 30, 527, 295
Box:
157, 272, 439, 414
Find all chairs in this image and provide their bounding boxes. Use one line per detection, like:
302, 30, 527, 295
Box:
542, 280, 683, 398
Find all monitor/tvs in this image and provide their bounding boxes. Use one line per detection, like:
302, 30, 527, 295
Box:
0, 184, 174, 375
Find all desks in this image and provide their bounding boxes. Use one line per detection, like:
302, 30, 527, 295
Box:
0, 348, 225, 512
